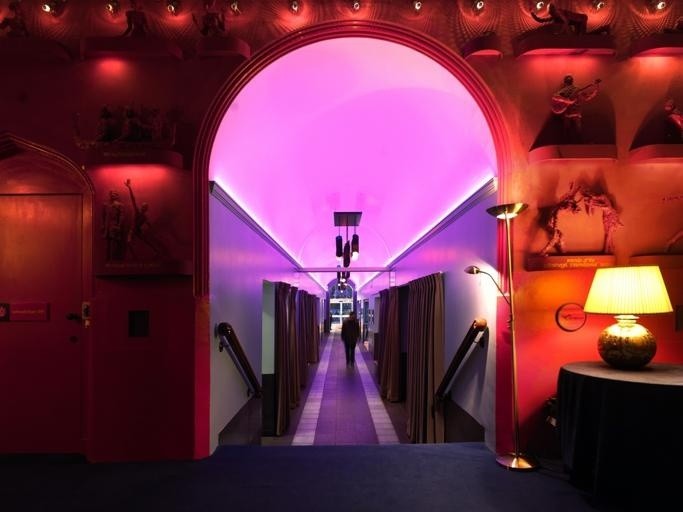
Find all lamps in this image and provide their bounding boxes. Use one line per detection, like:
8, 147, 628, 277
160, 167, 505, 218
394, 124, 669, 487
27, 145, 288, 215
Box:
333, 212, 361, 293
464, 203, 540, 473
583, 265, 675, 370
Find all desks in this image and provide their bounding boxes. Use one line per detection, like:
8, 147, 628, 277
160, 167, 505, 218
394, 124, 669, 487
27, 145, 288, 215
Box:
555, 363, 683, 512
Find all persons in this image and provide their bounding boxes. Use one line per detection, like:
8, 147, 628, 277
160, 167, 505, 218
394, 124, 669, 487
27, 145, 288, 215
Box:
340, 311, 360, 367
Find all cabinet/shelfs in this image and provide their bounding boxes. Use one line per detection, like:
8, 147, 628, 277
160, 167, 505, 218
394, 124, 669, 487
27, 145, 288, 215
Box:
79, 142, 183, 278
514, 28, 683, 271
